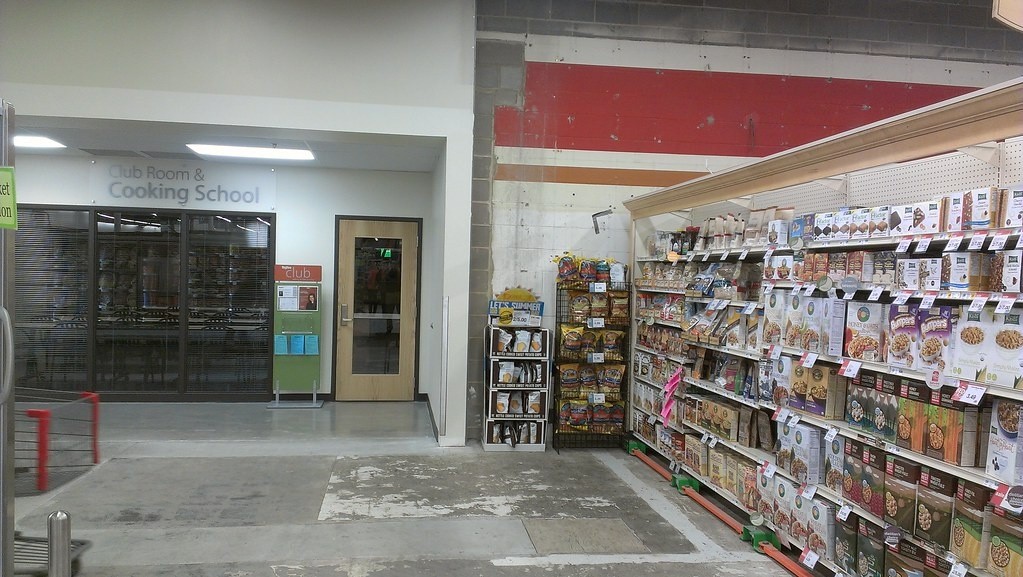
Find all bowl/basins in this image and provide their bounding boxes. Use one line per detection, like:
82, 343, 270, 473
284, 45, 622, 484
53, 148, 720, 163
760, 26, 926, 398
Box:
922, 347, 941, 362
714, 423, 721, 429
705, 418, 711, 424
811, 386, 827, 406
997, 345, 1020, 361
792, 383, 806, 399
892, 342, 910, 357
723, 427, 730, 432
960, 330, 984, 355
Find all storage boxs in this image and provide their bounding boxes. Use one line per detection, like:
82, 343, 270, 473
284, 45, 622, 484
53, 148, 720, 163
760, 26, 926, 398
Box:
632, 188, 1023, 577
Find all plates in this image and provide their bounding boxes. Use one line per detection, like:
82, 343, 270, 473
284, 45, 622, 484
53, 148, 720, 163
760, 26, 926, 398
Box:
774, 511, 790, 527
758, 499, 774, 519
847, 336, 880, 360
792, 521, 803, 538
997, 402, 1018, 434
808, 533, 827, 556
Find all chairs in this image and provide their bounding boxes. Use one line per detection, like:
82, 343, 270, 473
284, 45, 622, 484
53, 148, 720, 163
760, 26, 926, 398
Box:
30, 302, 270, 392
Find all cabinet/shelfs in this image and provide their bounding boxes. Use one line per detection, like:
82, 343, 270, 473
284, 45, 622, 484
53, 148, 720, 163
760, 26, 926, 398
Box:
479, 314, 549, 452
553, 282, 631, 455
188, 246, 269, 307
48, 234, 180, 307
628, 134, 1023, 577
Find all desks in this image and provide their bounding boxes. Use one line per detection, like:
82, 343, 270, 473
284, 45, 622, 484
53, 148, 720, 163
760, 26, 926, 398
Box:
46, 318, 268, 325
13, 325, 263, 380
97, 312, 266, 318
134, 307, 268, 311
